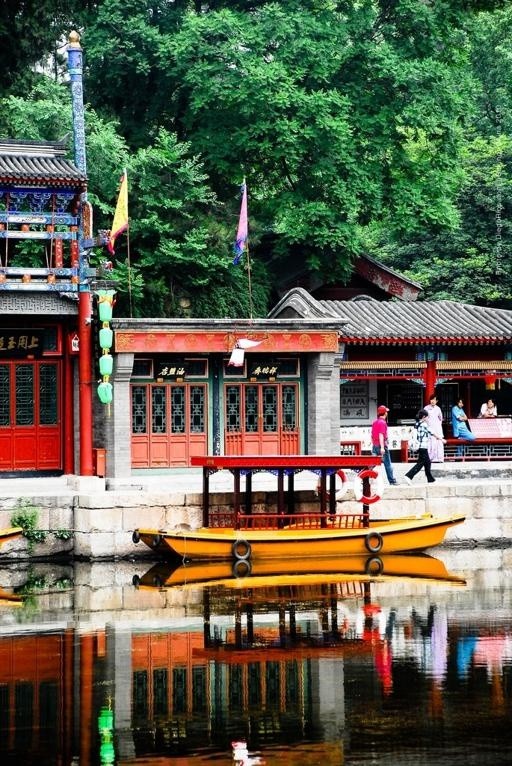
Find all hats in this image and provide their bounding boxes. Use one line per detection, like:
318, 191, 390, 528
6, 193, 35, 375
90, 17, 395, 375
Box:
377, 405, 390, 414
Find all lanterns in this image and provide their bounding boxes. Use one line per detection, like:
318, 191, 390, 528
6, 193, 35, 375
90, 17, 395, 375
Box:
97, 381, 114, 405
99, 354, 113, 375
99, 297, 112, 321
99, 327, 114, 348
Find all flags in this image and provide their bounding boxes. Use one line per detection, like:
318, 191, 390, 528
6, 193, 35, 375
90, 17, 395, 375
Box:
233, 182, 247, 265
108, 172, 127, 249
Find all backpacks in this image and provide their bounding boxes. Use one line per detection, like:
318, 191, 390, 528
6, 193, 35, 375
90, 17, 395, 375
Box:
407, 421, 427, 452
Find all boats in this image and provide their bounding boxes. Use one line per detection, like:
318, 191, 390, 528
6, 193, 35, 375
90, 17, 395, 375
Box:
133, 456, 466, 562
132, 553, 466, 663
1, 587, 24, 607
1, 527, 23, 549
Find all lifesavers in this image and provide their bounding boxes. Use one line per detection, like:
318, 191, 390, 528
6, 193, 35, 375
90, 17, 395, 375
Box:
315, 469, 348, 502
353, 469, 385, 504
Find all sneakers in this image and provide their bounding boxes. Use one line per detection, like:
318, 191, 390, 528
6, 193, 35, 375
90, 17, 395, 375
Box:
389, 480, 400, 487
427, 479, 442, 487
402, 474, 413, 486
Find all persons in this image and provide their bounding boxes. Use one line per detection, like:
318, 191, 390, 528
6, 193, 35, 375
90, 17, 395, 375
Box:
451, 398, 475, 456
422, 393, 447, 463
477, 397, 497, 418
371, 610, 510, 720
367, 405, 401, 486
403, 409, 437, 486
